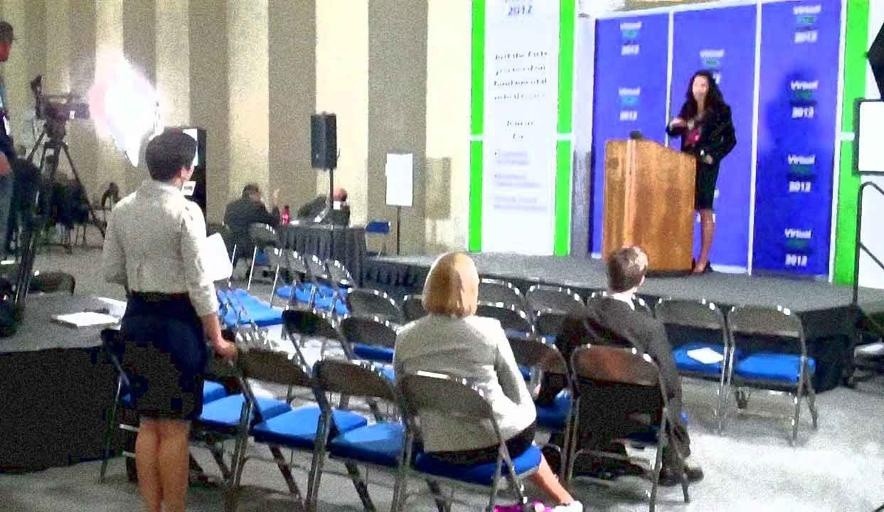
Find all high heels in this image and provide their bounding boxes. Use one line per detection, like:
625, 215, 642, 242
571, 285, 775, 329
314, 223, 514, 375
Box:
691, 260, 710, 275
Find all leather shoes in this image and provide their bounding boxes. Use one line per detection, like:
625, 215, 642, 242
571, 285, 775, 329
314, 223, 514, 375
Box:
658, 466, 703, 486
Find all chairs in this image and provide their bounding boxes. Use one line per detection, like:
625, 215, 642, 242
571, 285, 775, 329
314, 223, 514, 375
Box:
395, 368, 543, 512
247, 222, 397, 320
533, 305, 573, 334
526, 284, 586, 315
214, 272, 286, 350
586, 293, 652, 323
97, 326, 223, 490
235, 345, 367, 510
402, 294, 429, 322
197, 342, 293, 511
722, 302, 817, 446
567, 342, 693, 512
309, 354, 424, 511
473, 297, 532, 335
283, 302, 352, 407
340, 310, 397, 423
654, 292, 731, 434
502, 332, 575, 493
479, 279, 525, 310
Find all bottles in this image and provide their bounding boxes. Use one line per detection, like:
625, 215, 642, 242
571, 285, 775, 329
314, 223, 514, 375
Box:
282, 206, 289, 226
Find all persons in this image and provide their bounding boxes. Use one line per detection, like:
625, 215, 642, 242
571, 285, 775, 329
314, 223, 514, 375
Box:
297, 188, 350, 225
224, 184, 280, 279
665, 70, 737, 275
103, 128, 238, 512
0, 21, 19, 261
393, 252, 583, 512
532, 246, 704, 486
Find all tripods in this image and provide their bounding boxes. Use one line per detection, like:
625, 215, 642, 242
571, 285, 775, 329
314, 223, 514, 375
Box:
9, 141, 106, 318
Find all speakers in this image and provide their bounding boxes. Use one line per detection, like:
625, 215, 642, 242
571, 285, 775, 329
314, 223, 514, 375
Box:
310, 113, 338, 170
383, 152, 414, 207
